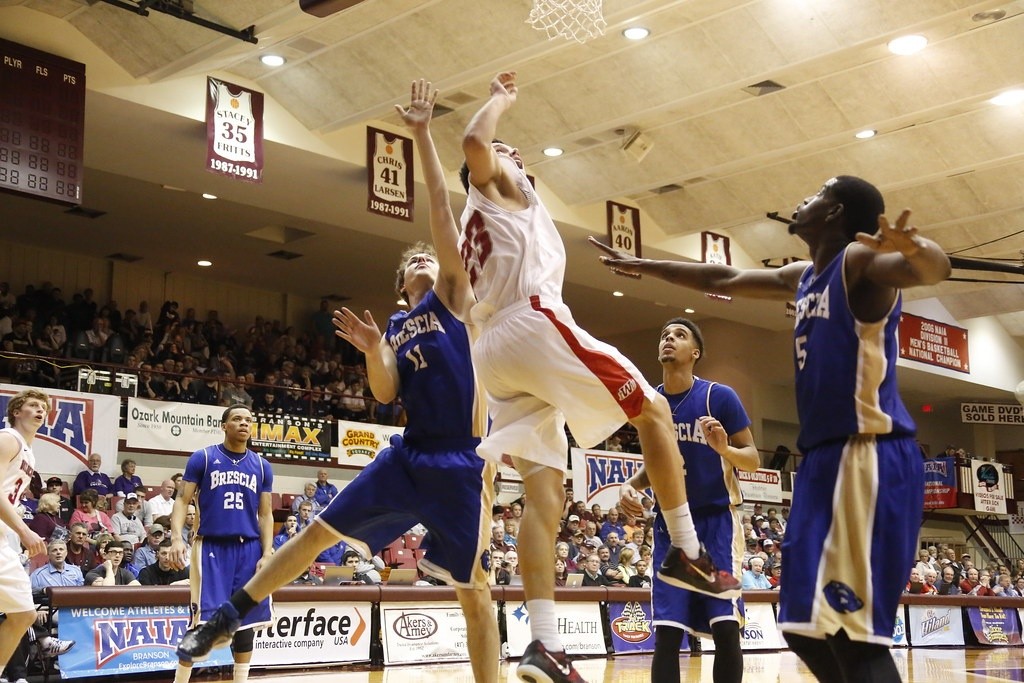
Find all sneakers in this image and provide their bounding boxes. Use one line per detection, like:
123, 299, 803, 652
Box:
41, 637, 76, 657
518, 640, 589, 683
176, 601, 241, 661
657, 541, 742, 599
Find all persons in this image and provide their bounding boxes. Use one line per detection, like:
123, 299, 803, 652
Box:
0, 279, 1024, 626
0, 610, 75, 683
456, 68, 741, 683
174, 78, 499, 683
619, 316, 760, 683
0, 390, 51, 677
590, 176, 951, 683
168, 402, 274, 683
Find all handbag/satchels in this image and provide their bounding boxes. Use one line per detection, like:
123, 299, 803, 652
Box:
49, 526, 71, 542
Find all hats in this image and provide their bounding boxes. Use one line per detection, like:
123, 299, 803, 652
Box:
756, 515, 765, 522
762, 539, 773, 547
569, 515, 581, 523
573, 529, 583, 538
124, 493, 138, 502
771, 563, 781, 569
577, 554, 589, 562
46, 477, 62, 485
754, 503, 762, 508
581, 540, 596, 549
150, 523, 164, 535
783, 507, 789, 513
941, 559, 952, 565
961, 553, 971, 558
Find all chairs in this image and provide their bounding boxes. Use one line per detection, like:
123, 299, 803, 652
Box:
23, 485, 431, 584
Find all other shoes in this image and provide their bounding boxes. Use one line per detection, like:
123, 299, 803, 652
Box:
16, 678, 29, 683
0, 678, 9, 683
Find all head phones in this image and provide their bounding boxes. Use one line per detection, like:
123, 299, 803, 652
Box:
748, 556, 766, 571
941, 567, 957, 579
966, 567, 980, 580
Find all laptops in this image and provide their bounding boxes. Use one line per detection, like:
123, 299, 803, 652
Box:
564, 574, 584, 587
322, 567, 354, 586
387, 569, 417, 585
909, 582, 923, 594
938, 583, 950, 595
509, 575, 522, 586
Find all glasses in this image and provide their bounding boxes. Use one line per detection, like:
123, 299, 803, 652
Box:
107, 550, 125, 556
89, 459, 100, 462
